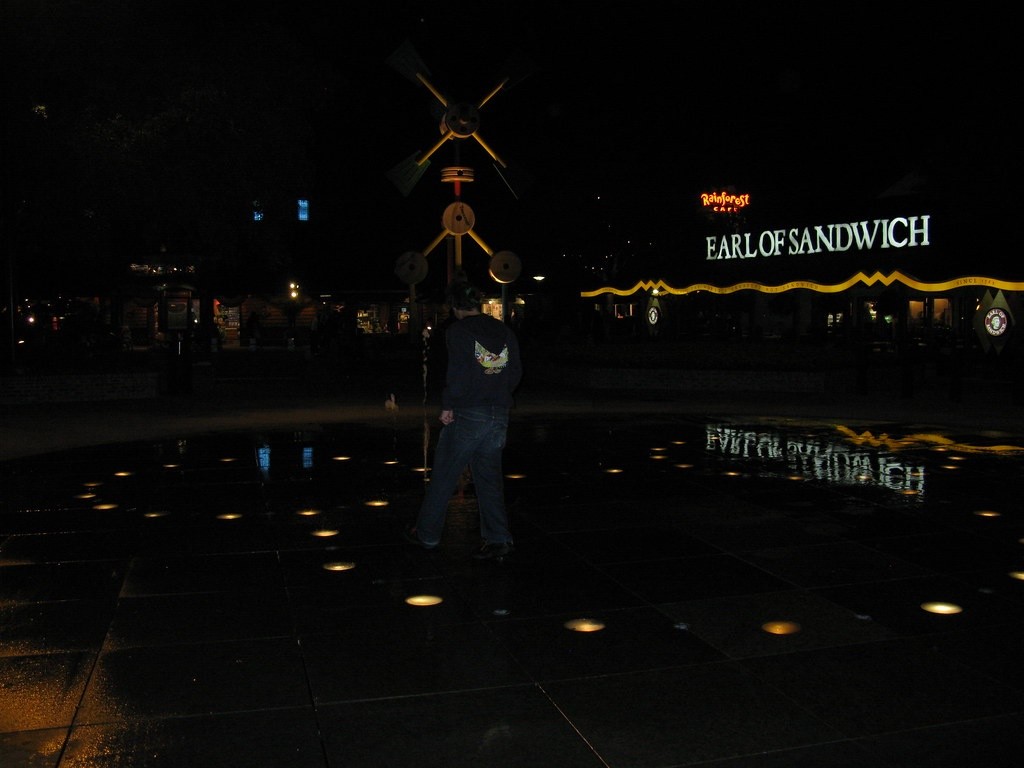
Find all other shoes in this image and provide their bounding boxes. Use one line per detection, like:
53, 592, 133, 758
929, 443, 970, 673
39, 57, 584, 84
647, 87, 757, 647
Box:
404, 523, 437, 548
473, 542, 511, 560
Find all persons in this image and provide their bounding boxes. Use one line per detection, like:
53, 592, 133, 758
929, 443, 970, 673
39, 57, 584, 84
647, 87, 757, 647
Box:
404, 278, 522, 560
246, 310, 261, 352
309, 312, 321, 359
